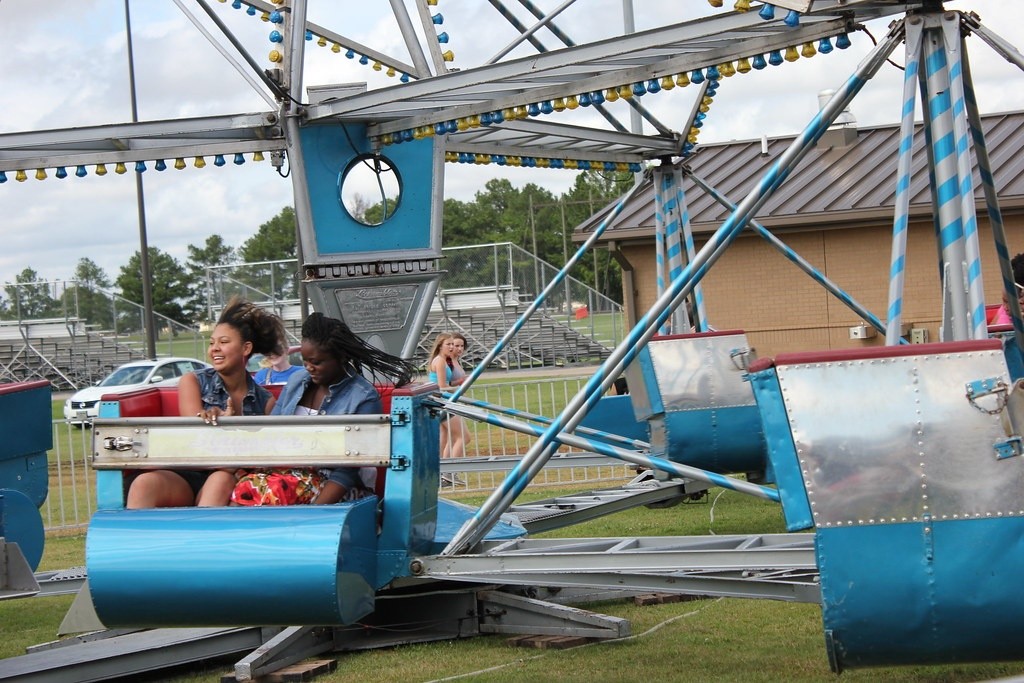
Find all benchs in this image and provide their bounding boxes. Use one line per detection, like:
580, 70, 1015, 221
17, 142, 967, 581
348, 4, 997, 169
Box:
92, 381, 441, 507
0, 332, 145, 393
281, 304, 612, 369
0, 379, 53, 508
742, 337, 1024, 515
623, 329, 757, 420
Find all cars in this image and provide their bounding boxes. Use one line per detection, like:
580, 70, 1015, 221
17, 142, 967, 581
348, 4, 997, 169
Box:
64, 357, 214, 430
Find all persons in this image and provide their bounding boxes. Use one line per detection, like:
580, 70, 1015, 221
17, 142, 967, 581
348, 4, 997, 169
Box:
427, 332, 471, 487
126, 299, 290, 509
1000, 252, 1024, 386
254, 341, 305, 385
229, 312, 422, 507
